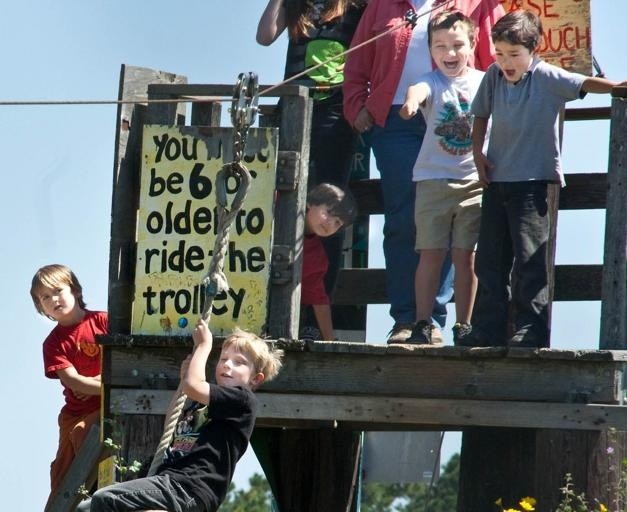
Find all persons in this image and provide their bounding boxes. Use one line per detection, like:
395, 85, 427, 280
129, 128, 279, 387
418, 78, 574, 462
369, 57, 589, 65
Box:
253, 1, 371, 340
299, 179, 358, 344
85, 316, 287, 512
468, 8, 625, 349
397, 8, 487, 345
341, 1, 508, 344
25, 262, 110, 512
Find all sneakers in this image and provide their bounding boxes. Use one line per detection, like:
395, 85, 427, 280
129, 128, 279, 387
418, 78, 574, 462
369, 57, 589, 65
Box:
506, 329, 547, 347
460, 325, 510, 345
452, 323, 473, 348
387, 321, 442, 345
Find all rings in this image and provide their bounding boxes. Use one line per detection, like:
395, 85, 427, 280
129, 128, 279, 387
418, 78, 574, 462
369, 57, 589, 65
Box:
363, 124, 368, 131
